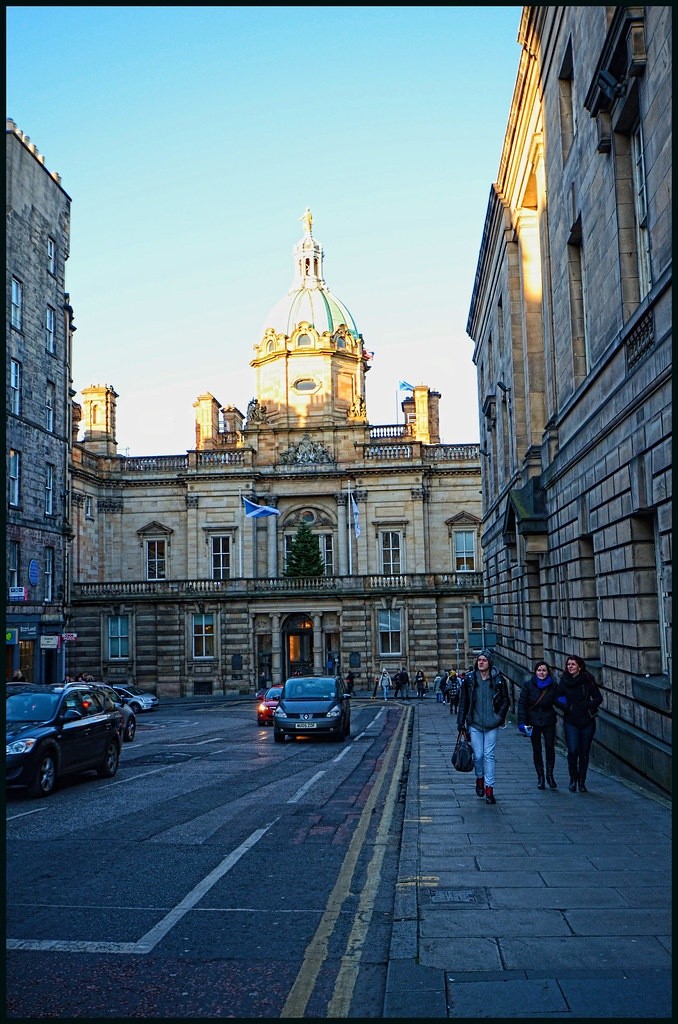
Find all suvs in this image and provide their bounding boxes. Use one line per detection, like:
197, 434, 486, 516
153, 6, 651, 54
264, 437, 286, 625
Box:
67, 681, 136, 743
6, 682, 125, 798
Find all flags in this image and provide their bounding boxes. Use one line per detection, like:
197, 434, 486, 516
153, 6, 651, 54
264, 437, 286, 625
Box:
351, 494, 361, 538
243, 497, 281, 517
399, 381, 414, 391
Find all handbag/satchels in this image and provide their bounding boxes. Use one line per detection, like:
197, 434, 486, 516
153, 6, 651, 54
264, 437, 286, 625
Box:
346, 675, 350, 682
584, 681, 600, 720
451, 729, 475, 772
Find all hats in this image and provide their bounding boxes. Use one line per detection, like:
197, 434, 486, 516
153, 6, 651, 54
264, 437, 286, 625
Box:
383, 667, 406, 673
444, 669, 466, 676
473, 647, 495, 688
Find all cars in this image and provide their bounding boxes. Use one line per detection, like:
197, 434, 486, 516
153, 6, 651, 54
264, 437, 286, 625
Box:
112, 683, 160, 714
273, 675, 352, 743
256, 684, 284, 726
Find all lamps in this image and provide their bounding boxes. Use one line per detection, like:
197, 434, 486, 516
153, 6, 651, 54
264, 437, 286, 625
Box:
496, 381, 511, 392
478, 450, 491, 457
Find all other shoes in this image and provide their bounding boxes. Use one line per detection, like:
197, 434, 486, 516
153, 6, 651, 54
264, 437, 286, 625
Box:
354, 694, 356, 696
501, 725, 507, 730
385, 698, 424, 701
436, 699, 450, 705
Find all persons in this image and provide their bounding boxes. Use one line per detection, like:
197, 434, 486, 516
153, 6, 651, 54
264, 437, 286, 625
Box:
327, 661, 475, 714
285, 441, 333, 462
556, 655, 603, 792
252, 399, 266, 421
299, 208, 313, 231
519, 661, 568, 789
458, 649, 510, 803
13, 669, 25, 682
63, 672, 94, 683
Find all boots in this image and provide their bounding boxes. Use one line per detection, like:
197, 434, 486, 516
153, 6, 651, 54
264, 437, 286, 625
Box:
476, 776, 485, 797
546, 768, 557, 788
536, 769, 545, 789
484, 787, 496, 804
569, 767, 587, 792
450, 709, 457, 714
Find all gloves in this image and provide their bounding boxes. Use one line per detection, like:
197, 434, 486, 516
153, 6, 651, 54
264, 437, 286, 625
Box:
558, 697, 567, 704
458, 725, 466, 735
519, 723, 526, 732
